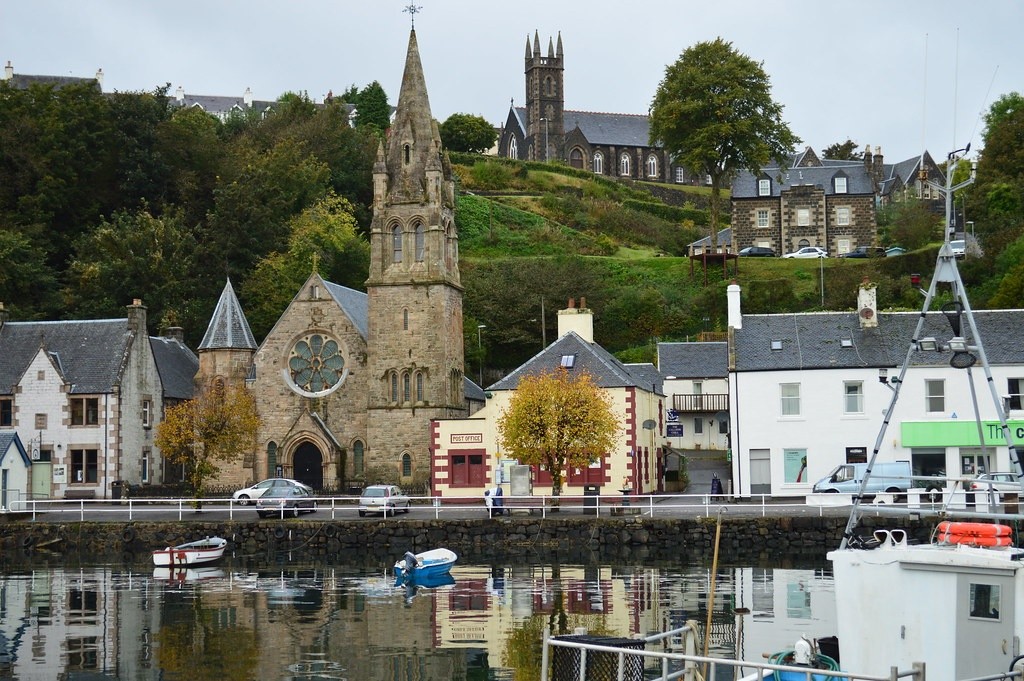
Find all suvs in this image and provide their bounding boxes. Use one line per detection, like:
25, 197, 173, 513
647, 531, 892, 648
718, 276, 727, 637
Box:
969, 473, 1024, 502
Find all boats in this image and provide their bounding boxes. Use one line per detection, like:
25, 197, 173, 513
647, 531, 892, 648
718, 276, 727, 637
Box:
547, 160, 1024, 681
393, 573, 456, 598
153, 567, 224, 585
394, 549, 458, 578
151, 536, 227, 566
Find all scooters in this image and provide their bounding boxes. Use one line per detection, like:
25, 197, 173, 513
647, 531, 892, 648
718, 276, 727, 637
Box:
485, 489, 503, 516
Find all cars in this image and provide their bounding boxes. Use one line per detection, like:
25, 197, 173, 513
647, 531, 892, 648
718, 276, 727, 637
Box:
359, 484, 410, 517
836, 247, 887, 258
951, 240, 965, 258
256, 486, 317, 518
736, 247, 775, 256
780, 247, 830, 259
232, 479, 313, 506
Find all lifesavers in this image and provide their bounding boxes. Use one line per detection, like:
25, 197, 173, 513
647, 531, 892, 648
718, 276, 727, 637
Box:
935, 519, 1014, 549
325, 524, 336, 538
122, 529, 135, 544
23, 536, 33, 548
273, 525, 286, 539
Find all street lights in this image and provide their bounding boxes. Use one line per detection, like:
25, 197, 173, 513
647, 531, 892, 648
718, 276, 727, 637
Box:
967, 221, 975, 238
479, 325, 486, 385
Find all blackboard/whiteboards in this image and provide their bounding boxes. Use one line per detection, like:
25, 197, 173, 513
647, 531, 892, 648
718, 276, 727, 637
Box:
711, 478, 721, 496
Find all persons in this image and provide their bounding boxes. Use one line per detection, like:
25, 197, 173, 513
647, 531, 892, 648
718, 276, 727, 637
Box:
793, 632, 817, 670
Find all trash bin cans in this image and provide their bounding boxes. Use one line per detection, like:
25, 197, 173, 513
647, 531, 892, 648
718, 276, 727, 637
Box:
111, 480, 130, 506
583, 483, 600, 514
1003, 493, 1019, 514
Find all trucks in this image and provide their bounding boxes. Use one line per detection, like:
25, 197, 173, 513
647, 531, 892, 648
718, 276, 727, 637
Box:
813, 460, 912, 502
